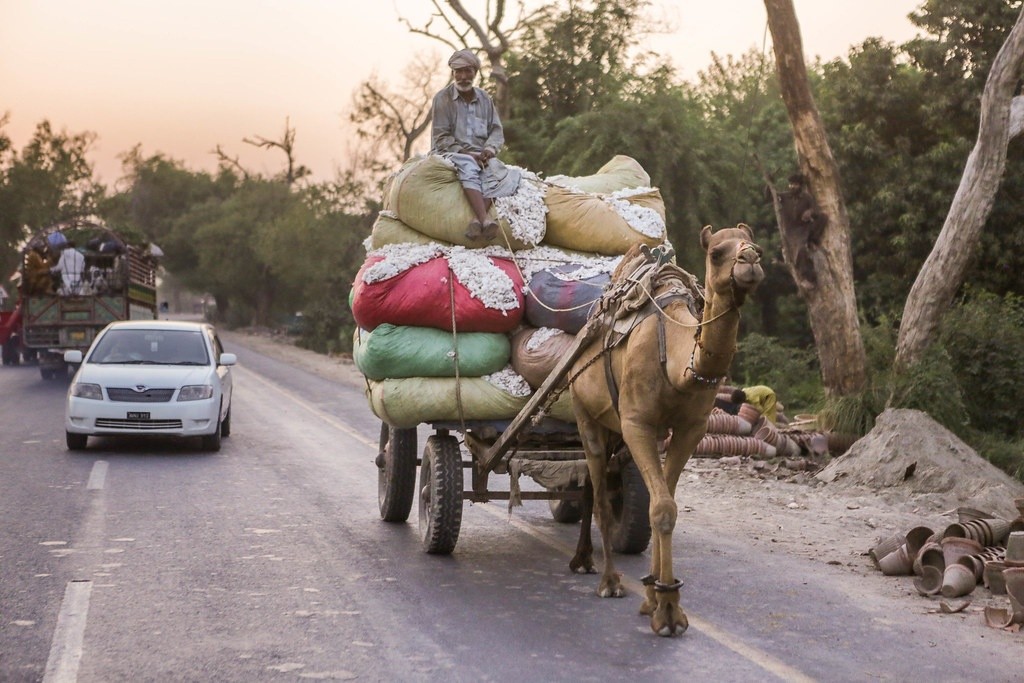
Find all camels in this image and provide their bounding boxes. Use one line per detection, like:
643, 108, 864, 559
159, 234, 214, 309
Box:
567, 223, 764, 637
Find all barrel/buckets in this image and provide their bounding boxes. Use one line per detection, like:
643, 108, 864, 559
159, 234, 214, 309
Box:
659, 385, 832, 457
871, 500, 1024, 630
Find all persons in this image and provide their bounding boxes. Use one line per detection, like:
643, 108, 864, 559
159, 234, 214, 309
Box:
103, 336, 142, 363
26, 239, 126, 297
430, 49, 504, 241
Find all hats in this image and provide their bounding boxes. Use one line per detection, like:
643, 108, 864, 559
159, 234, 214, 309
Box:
448, 49, 481, 69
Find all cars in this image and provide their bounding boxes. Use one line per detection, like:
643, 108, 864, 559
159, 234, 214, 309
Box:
64, 320, 238, 453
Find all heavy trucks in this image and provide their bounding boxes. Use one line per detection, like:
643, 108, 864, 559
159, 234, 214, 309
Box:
20, 243, 170, 380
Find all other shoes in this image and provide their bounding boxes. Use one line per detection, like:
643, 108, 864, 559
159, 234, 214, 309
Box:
484, 223, 499, 240
464, 221, 482, 241
